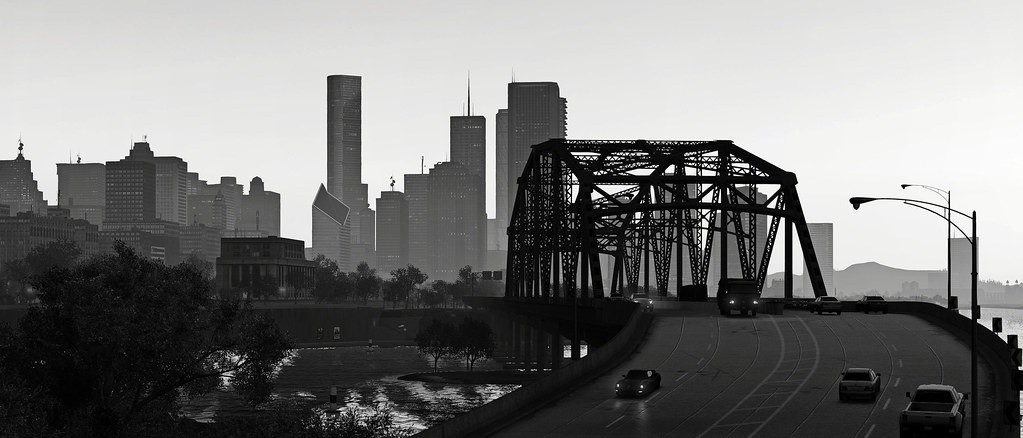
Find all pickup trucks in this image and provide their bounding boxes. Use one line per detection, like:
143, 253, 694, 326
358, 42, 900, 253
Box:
899, 385, 968, 438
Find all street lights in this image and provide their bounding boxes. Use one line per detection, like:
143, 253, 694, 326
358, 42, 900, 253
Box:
849, 196, 978, 438
901, 183, 951, 310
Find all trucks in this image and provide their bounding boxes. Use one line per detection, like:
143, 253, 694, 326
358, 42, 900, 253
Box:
676, 284, 708, 302
716, 278, 759, 316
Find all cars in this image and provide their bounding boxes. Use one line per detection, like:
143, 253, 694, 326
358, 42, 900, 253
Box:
611, 293, 623, 299
615, 369, 661, 398
839, 367, 881, 402
630, 294, 653, 310
809, 296, 843, 316
857, 295, 889, 314
